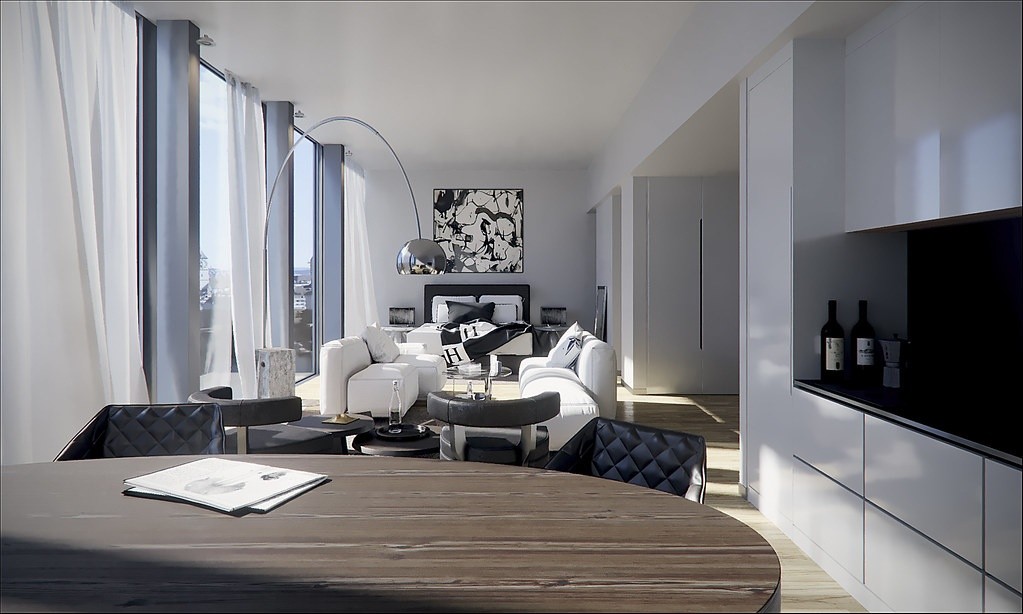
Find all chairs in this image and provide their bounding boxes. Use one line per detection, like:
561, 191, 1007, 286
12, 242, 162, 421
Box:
188, 386, 332, 456
545, 417, 707, 508
53, 404, 226, 461
427, 392, 560, 464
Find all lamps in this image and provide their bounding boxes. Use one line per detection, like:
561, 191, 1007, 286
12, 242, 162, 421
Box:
260, 116, 445, 348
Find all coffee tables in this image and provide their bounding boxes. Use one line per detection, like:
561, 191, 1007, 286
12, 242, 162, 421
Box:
353, 425, 440, 457
286, 414, 374, 455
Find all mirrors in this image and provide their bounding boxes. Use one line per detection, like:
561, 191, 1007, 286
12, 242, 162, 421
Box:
594, 286, 606, 341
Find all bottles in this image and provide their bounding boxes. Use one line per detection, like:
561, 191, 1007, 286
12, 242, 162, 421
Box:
850, 300, 876, 388
820, 299, 845, 386
388, 380, 402, 434
467, 382, 472, 398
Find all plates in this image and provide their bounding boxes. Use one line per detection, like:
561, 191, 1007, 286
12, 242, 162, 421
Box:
375, 423, 431, 441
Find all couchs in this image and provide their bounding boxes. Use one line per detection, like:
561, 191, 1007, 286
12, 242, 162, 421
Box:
518, 330, 617, 451
320, 336, 448, 424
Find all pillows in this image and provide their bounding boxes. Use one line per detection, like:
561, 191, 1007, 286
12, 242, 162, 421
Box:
548, 322, 583, 367
360, 322, 401, 362
446, 300, 496, 324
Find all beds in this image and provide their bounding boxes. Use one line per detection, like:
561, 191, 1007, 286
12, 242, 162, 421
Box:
407, 284, 533, 356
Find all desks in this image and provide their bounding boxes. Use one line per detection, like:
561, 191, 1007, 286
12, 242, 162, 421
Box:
443, 365, 512, 396
0, 454, 782, 614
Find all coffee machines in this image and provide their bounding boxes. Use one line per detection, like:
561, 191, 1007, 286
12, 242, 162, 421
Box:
878, 332, 912, 392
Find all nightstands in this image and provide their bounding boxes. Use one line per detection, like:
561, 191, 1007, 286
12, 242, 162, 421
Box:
383, 327, 413, 342
534, 326, 569, 357
